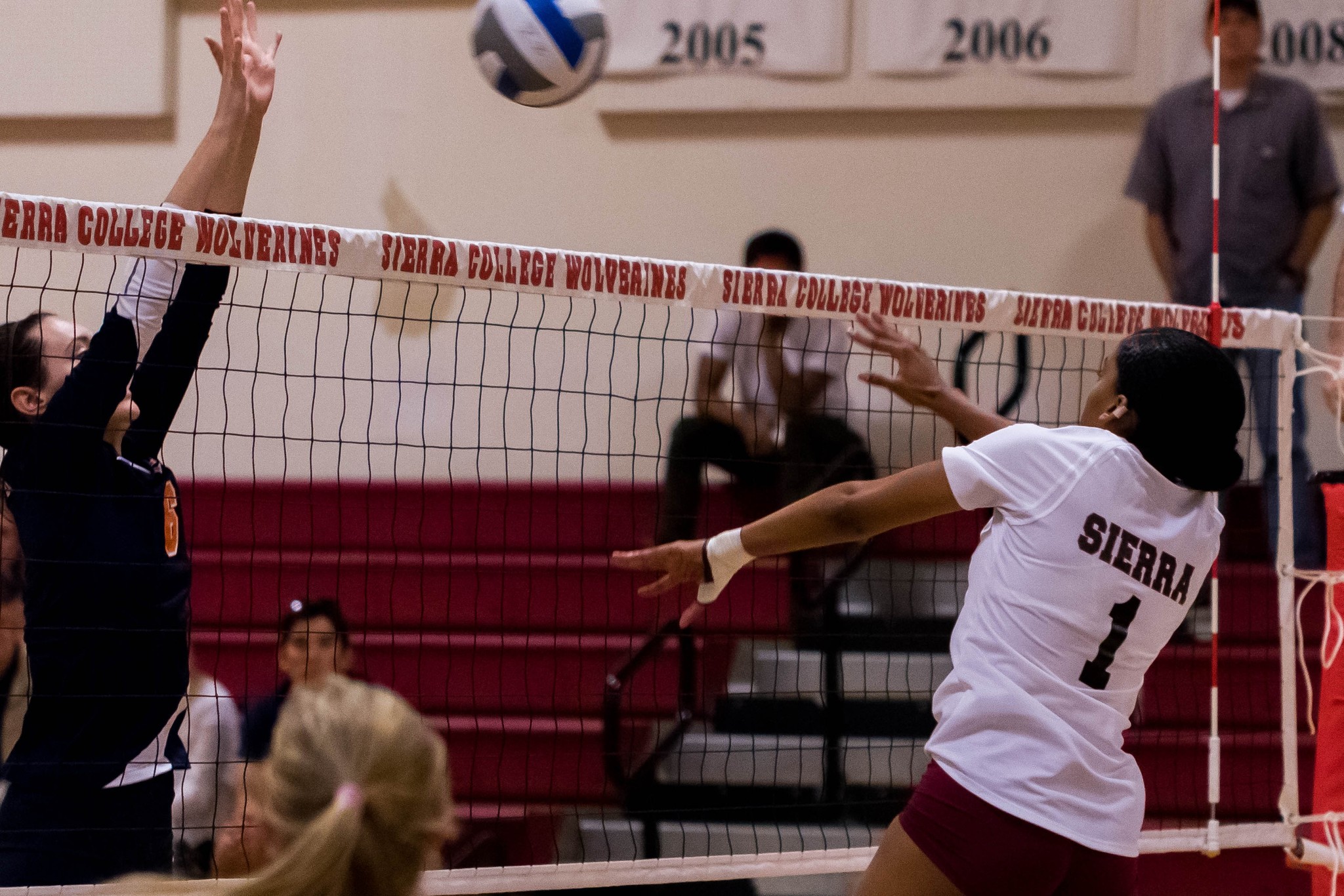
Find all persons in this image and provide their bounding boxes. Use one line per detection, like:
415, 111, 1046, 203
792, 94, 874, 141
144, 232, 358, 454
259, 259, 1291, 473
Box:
652, 227, 881, 552
611, 312, 1254, 893
0, 1, 283, 877
1126, 1, 1344, 564
0, 568, 459, 896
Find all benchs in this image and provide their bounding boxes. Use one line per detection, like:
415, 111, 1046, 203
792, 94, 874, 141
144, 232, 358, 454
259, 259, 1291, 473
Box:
172, 476, 1344, 896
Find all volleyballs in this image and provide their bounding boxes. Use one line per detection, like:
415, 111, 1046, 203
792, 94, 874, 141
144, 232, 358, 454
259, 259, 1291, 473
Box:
471, 0, 611, 108
